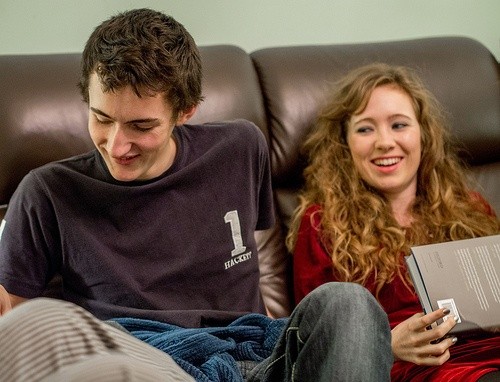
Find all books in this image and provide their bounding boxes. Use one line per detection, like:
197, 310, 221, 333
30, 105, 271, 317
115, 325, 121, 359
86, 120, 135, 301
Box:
404, 235, 500, 345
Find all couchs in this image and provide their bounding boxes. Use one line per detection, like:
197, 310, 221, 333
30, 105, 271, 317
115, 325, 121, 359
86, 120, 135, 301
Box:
1, 37, 500, 319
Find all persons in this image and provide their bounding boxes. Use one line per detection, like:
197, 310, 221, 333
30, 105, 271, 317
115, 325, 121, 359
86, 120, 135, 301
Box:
293, 62, 500, 382
0, 9, 393, 382
